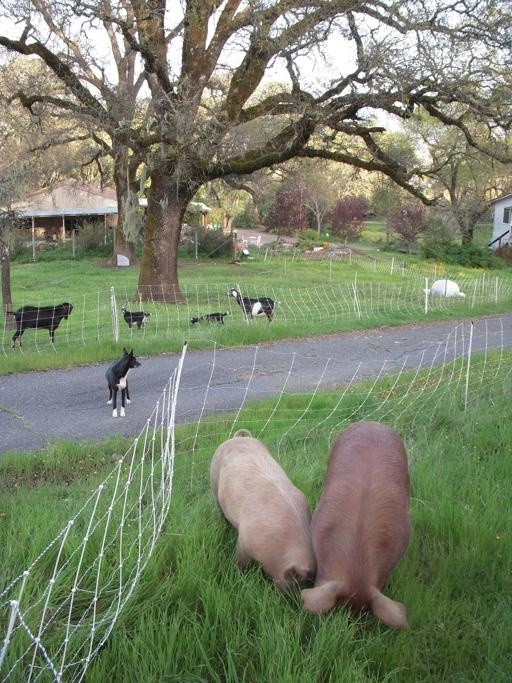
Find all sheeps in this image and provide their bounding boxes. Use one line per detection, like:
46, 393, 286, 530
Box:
189, 312, 228, 325
227, 288, 277, 325
120, 306, 151, 330
5, 302, 74, 351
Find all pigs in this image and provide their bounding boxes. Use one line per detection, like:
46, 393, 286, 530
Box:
209, 429, 317, 597
298, 419, 408, 631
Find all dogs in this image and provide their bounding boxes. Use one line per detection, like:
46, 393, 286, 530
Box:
104, 347, 142, 418
